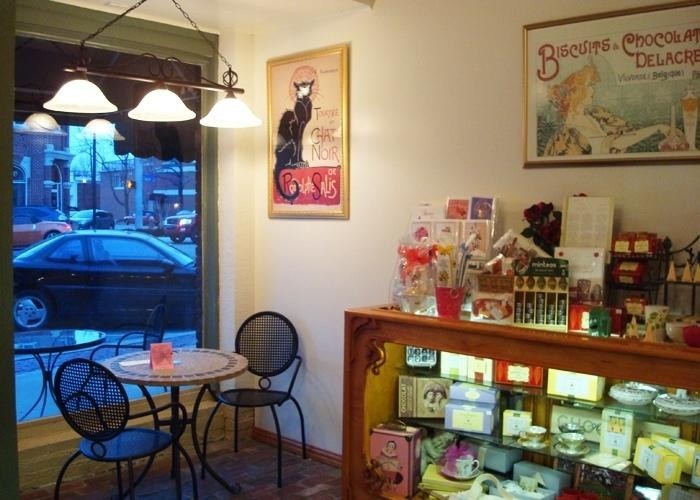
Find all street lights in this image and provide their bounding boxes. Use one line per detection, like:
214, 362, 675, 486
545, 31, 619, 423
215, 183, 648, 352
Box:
44, 157, 74, 212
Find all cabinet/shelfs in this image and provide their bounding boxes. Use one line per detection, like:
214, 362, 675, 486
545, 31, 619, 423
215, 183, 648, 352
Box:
342, 304, 700, 499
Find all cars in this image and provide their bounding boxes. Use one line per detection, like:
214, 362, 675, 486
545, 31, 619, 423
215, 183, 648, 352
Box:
10, 214, 71, 248
68, 209, 114, 230
124, 211, 160, 225
11, 230, 200, 330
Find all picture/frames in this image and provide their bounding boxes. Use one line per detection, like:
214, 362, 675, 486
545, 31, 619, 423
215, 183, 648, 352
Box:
266, 42, 350, 220
522, 0, 700, 169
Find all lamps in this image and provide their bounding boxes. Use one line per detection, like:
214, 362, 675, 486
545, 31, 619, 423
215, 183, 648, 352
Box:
42, 0, 263, 128
20, 109, 128, 141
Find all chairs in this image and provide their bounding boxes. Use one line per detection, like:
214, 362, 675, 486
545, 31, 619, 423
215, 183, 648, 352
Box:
201, 311, 307, 488
77, 303, 169, 409
54, 358, 198, 499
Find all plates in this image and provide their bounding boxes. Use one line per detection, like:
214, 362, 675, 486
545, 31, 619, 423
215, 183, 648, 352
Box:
553, 441, 591, 457
440, 466, 479, 481
517, 437, 551, 449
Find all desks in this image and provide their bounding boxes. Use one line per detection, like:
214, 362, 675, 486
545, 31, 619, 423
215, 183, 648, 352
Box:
98, 348, 249, 481
12, 329, 106, 422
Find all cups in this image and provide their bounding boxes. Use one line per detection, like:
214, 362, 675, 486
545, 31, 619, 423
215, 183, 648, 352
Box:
455, 454, 479, 478
518, 426, 547, 444
435, 286, 466, 320
645, 304, 669, 341
558, 432, 584, 451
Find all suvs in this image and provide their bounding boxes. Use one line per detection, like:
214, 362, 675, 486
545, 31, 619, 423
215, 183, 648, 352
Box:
162, 212, 199, 243
13, 206, 67, 221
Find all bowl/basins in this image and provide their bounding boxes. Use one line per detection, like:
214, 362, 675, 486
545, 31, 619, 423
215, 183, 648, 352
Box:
665, 321, 700, 344
